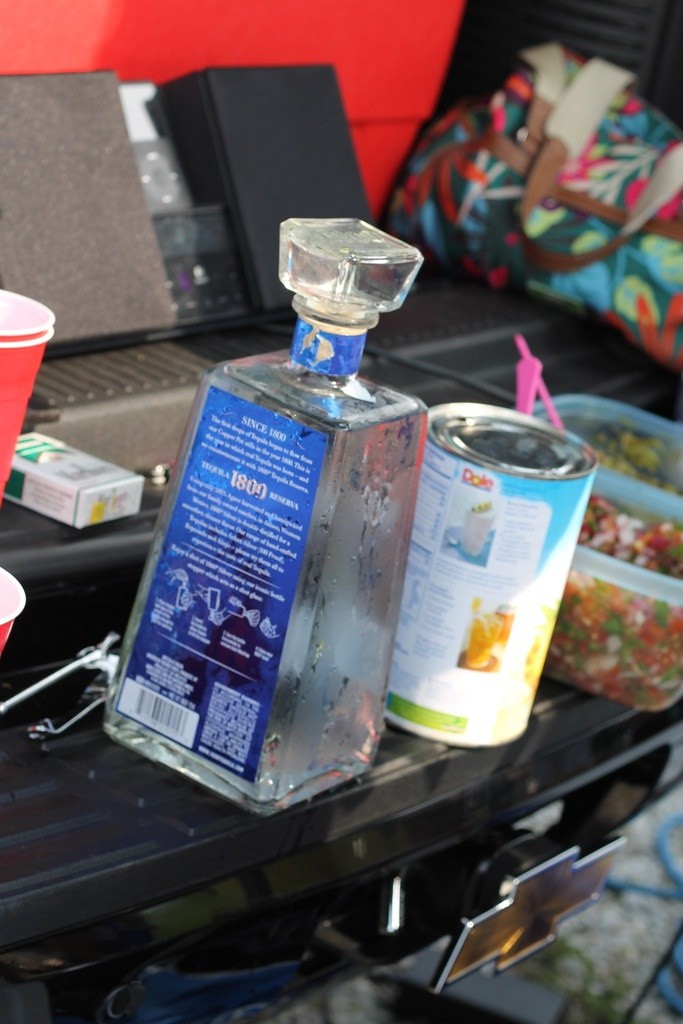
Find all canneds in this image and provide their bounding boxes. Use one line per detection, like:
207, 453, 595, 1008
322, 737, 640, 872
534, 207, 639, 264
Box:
388, 402, 597, 748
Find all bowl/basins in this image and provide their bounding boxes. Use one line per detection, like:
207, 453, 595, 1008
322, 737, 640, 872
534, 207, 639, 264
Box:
515, 395, 683, 712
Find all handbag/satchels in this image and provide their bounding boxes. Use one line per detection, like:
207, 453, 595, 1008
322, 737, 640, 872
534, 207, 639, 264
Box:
386, 39, 683, 372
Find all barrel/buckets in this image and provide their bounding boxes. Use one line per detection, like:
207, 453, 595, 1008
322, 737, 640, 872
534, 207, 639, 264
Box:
382, 402, 600, 748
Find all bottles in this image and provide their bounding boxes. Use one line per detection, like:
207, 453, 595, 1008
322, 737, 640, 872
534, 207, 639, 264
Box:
104, 217, 430, 816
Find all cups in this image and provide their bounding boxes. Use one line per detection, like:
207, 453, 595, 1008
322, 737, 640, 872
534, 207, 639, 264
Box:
0, 289, 56, 509
0, 567, 27, 662
